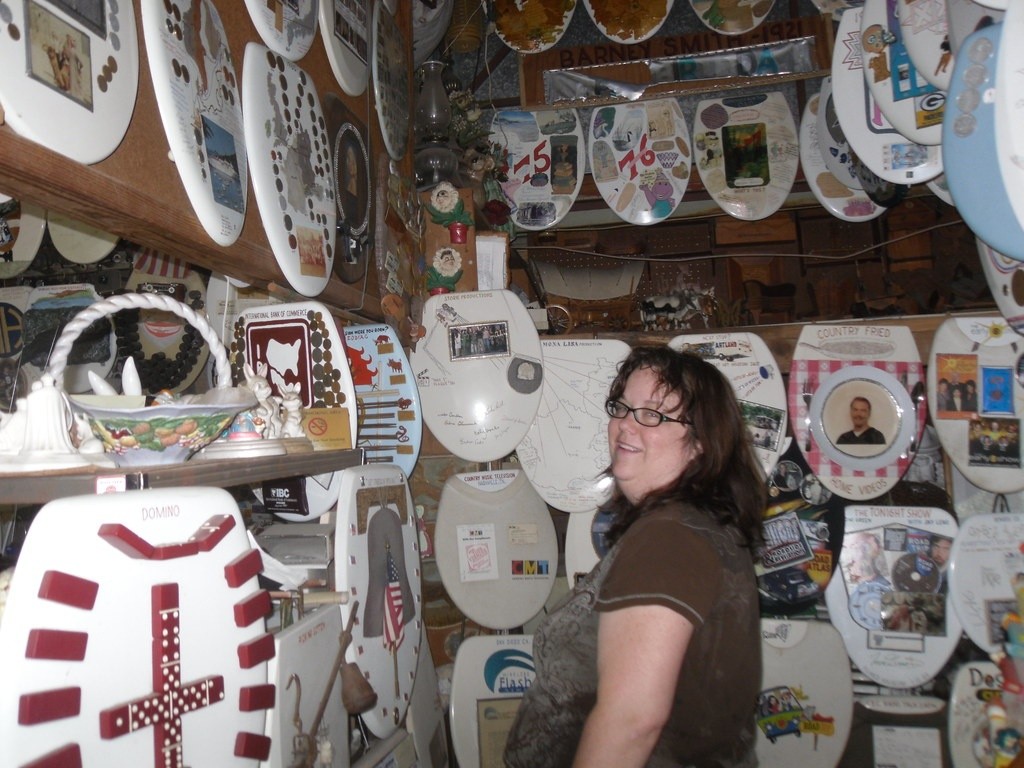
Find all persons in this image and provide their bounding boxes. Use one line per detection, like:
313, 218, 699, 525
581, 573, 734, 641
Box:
833, 397, 886, 445
502, 340, 774, 767
938, 376, 1019, 452
763, 462, 953, 634
450, 324, 510, 357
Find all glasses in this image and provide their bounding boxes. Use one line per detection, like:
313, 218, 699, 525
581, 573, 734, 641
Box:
605, 399, 694, 427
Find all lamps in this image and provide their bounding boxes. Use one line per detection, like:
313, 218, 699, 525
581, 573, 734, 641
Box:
411, 52, 457, 191
